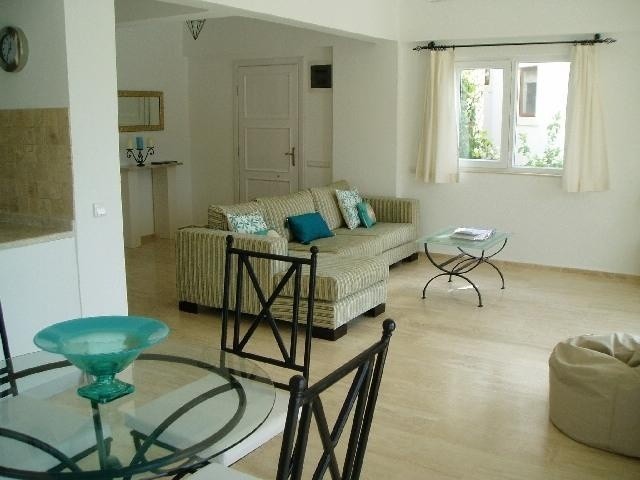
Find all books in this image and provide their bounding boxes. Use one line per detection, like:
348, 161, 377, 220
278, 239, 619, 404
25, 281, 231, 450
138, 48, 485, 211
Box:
448, 227, 496, 241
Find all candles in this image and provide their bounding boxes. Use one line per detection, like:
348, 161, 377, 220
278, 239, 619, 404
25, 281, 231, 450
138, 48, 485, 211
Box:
128, 136, 132, 148
147, 136, 152, 146
136, 136, 143, 148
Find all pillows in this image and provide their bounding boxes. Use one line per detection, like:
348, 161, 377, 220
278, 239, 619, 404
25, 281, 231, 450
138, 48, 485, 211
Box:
225, 208, 268, 235
253, 229, 282, 237
355, 201, 377, 229
286, 210, 335, 245
335, 184, 364, 231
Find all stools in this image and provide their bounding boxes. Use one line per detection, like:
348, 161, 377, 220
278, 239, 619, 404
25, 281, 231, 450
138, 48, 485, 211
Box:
547, 329, 640, 459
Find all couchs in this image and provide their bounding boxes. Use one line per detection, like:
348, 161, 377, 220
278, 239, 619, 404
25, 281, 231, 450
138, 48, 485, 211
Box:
173, 179, 419, 343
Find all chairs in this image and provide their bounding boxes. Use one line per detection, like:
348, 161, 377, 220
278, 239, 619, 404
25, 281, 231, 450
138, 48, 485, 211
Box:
175, 319, 396, 479
122, 234, 318, 479
1, 300, 113, 476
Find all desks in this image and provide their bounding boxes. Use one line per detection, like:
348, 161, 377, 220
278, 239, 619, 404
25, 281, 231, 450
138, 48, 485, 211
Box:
0, 340, 277, 480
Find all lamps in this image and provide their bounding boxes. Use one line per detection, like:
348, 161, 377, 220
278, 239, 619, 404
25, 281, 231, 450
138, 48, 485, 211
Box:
183, 15, 206, 42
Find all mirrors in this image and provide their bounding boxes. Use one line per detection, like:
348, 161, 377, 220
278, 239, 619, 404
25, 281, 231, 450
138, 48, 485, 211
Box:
117, 91, 165, 132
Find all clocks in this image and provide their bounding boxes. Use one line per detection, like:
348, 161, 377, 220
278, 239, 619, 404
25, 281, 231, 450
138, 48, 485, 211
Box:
0, 26, 30, 74
